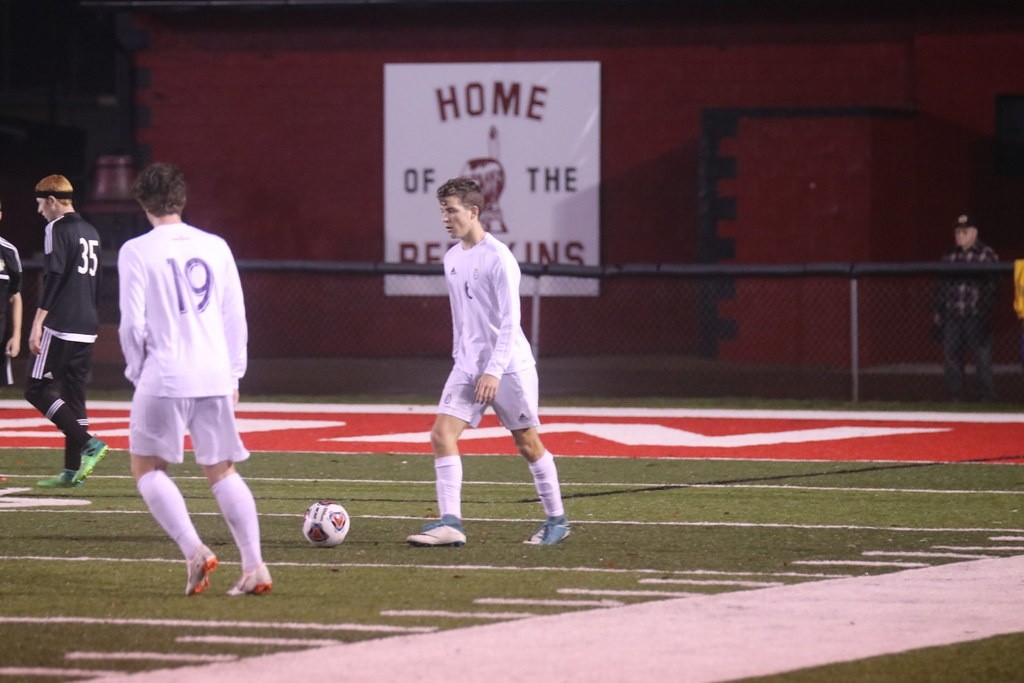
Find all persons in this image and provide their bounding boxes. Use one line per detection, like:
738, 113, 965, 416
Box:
23, 173, 111, 488
407, 178, 571, 545
118, 162, 274, 594
930, 211, 1000, 409
0, 201, 25, 386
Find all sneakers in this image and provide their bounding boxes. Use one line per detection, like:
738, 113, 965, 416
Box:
183, 544, 219, 596
36, 468, 85, 490
72, 436, 109, 489
521, 523, 572, 545
225, 562, 274, 597
405, 524, 468, 548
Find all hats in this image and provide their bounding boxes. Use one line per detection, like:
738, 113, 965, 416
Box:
947, 212, 980, 228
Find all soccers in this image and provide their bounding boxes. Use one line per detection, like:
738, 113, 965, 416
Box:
302, 500, 351, 548
